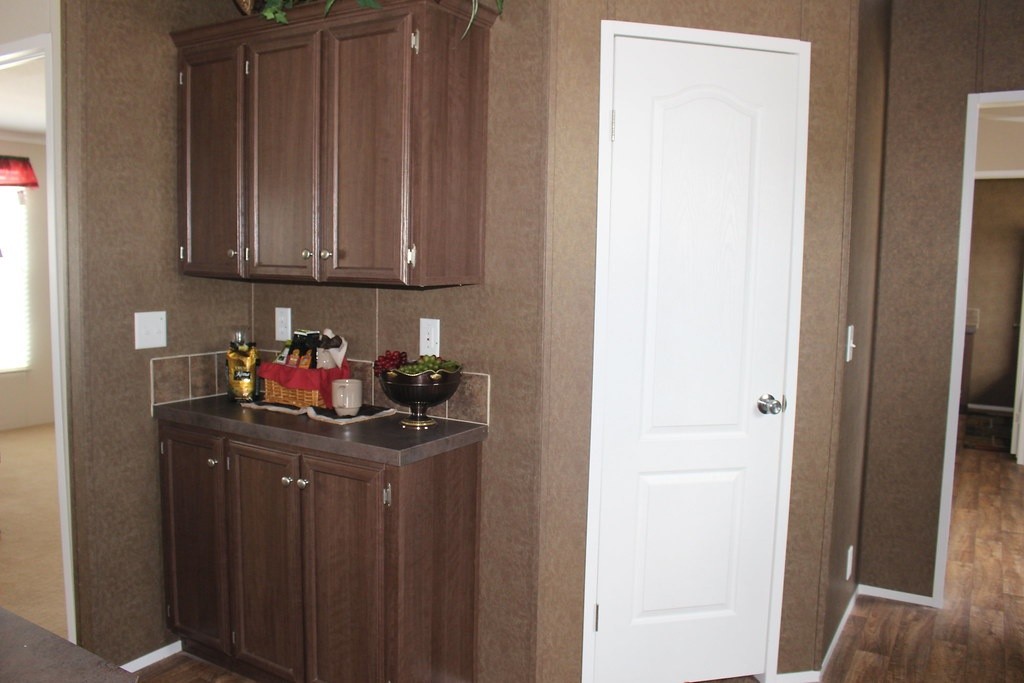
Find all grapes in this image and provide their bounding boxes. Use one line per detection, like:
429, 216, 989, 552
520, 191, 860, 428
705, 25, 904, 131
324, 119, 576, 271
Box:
373, 350, 447, 377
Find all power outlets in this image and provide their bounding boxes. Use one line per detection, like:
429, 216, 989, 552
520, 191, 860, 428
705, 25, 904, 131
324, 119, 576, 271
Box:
846, 545, 853, 580
275, 307, 292, 342
419, 318, 440, 357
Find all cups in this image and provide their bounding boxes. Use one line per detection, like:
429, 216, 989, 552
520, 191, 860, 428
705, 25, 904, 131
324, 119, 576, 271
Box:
331, 380, 362, 416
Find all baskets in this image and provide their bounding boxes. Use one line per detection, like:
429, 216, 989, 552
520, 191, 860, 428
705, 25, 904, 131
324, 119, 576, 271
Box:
260, 362, 352, 409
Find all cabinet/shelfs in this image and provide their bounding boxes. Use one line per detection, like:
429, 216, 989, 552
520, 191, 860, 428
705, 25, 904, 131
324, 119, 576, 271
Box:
176, 44, 244, 280
243, 13, 413, 287
158, 424, 233, 658
227, 439, 386, 683
